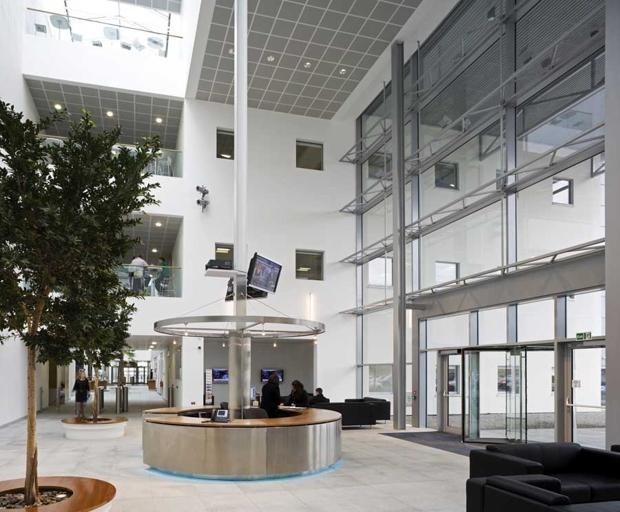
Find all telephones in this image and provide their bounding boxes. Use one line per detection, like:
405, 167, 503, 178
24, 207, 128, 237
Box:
211, 408, 229, 422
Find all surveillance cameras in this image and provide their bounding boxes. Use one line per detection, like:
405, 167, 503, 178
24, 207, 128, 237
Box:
197, 186, 208, 198
197, 199, 209, 212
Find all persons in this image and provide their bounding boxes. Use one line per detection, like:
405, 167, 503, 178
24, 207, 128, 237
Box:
294, 382, 309, 406
309, 388, 328, 404
73, 369, 91, 419
129, 253, 175, 298
287, 380, 298, 406
260, 372, 282, 417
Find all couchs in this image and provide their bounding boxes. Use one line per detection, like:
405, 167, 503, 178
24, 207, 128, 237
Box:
310, 397, 390, 428
466, 442, 620, 512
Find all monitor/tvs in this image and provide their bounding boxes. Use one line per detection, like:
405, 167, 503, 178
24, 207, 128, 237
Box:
212, 368, 228, 384
247, 252, 282, 295
261, 368, 283, 384
